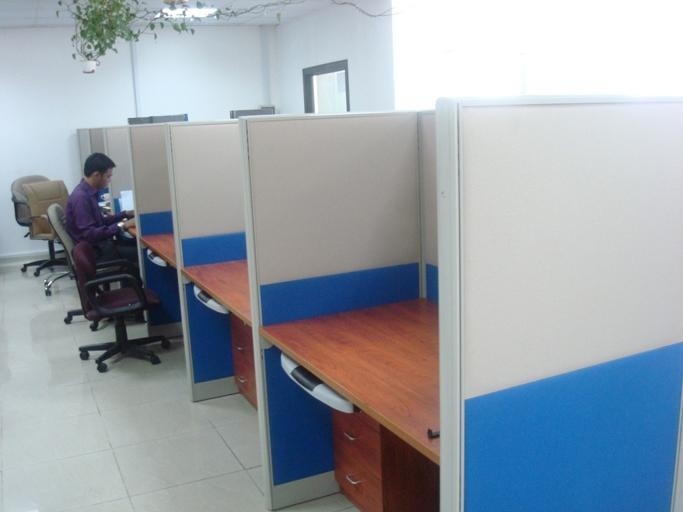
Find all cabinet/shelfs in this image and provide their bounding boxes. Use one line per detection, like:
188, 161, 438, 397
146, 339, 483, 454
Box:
321, 392, 405, 510
230, 309, 258, 412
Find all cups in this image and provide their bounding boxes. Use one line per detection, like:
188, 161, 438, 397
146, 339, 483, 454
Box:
100, 193, 110, 202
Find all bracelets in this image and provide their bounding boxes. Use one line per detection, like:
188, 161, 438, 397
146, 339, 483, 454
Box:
116, 221, 127, 232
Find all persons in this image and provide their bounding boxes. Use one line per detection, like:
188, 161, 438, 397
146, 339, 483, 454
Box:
62, 151, 144, 322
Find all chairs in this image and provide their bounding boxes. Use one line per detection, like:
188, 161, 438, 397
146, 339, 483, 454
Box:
10, 174, 124, 295
72, 242, 170, 372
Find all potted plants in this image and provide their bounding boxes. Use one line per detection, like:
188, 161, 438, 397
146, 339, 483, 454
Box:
56, 0, 236, 75
